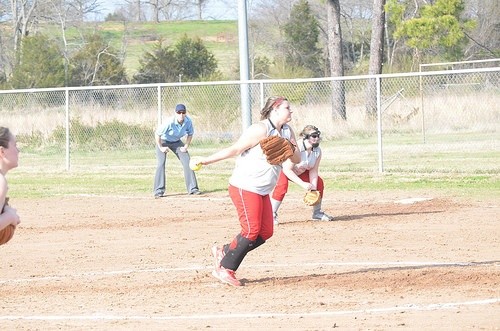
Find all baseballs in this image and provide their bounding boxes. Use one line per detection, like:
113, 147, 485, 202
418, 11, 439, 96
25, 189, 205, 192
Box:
188, 160, 203, 171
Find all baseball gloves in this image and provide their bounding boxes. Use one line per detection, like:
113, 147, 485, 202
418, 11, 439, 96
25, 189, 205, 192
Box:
0, 224, 16, 247
258, 136, 296, 166
303, 190, 321, 207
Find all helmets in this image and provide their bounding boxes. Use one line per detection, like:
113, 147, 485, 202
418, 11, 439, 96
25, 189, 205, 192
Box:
175, 104, 187, 111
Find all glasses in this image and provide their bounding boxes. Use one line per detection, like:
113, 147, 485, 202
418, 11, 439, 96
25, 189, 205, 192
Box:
311, 134, 321, 138
177, 111, 186, 114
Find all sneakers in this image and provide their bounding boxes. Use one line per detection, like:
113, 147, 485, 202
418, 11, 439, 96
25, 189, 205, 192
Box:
310, 211, 334, 221
212, 263, 242, 287
210, 244, 226, 270
272, 211, 279, 224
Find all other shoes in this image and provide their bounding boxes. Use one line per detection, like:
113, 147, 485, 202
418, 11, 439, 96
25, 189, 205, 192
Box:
155, 192, 164, 197
192, 189, 202, 194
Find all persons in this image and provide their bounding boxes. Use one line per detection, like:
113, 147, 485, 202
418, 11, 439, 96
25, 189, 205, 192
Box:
271, 124, 334, 224
0, 126, 21, 231
190, 96, 301, 286
153, 104, 201, 198
